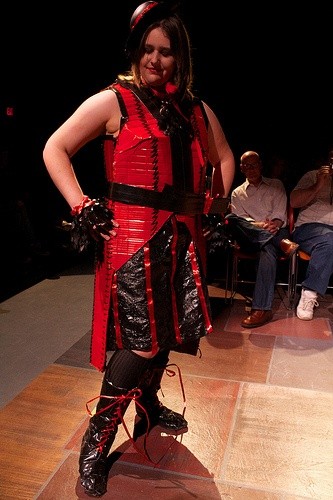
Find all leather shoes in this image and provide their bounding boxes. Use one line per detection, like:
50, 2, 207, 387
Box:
279, 237, 300, 257
242, 310, 264, 328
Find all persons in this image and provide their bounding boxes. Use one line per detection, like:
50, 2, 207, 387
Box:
43, 0, 234, 496
231, 150, 300, 329
290, 151, 333, 319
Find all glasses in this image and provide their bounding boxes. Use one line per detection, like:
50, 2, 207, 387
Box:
242, 160, 262, 169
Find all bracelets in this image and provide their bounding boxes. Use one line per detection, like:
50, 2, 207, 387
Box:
69, 195, 90, 215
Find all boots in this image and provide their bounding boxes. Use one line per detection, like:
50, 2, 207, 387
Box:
79, 377, 135, 496
135, 359, 188, 431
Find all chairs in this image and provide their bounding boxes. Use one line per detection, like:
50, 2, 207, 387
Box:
229, 207, 312, 318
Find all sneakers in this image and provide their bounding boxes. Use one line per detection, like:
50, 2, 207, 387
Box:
296, 289, 319, 320
